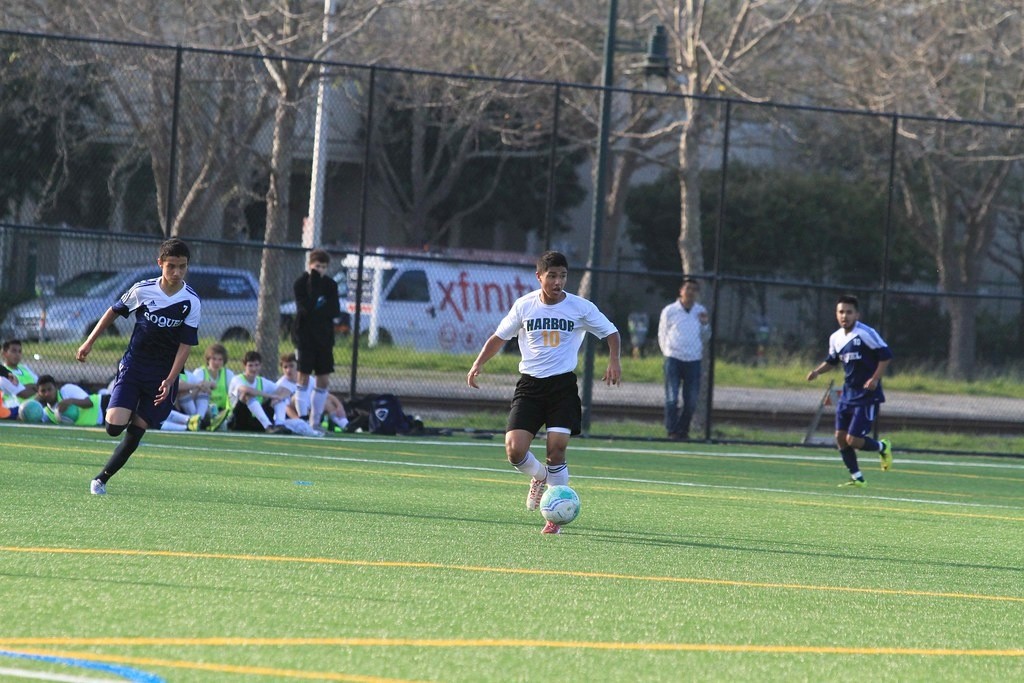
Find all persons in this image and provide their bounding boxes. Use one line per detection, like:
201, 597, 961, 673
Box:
290, 248, 342, 437
656, 278, 712, 440
74, 240, 202, 494
149, 345, 365, 432
0, 338, 114, 427
806, 293, 891, 488
466, 251, 622, 534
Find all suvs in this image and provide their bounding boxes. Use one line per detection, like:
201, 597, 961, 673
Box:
0, 264, 261, 345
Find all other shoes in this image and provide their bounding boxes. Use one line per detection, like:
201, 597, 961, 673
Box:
668, 433, 689, 442
91, 480, 106, 495
309, 427, 334, 437
344, 415, 363, 432
265, 426, 291, 434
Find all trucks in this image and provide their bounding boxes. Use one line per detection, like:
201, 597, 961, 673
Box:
280, 251, 543, 355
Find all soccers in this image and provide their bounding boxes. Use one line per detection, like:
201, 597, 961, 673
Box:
540, 484, 580, 526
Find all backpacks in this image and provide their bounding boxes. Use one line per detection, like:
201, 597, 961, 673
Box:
369, 394, 398, 435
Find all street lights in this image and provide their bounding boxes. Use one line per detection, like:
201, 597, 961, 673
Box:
580, 0, 672, 437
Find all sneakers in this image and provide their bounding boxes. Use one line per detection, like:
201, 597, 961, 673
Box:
542, 521, 560, 534
838, 480, 868, 489
187, 414, 201, 431
206, 410, 229, 431
526, 466, 549, 511
880, 439, 892, 472
